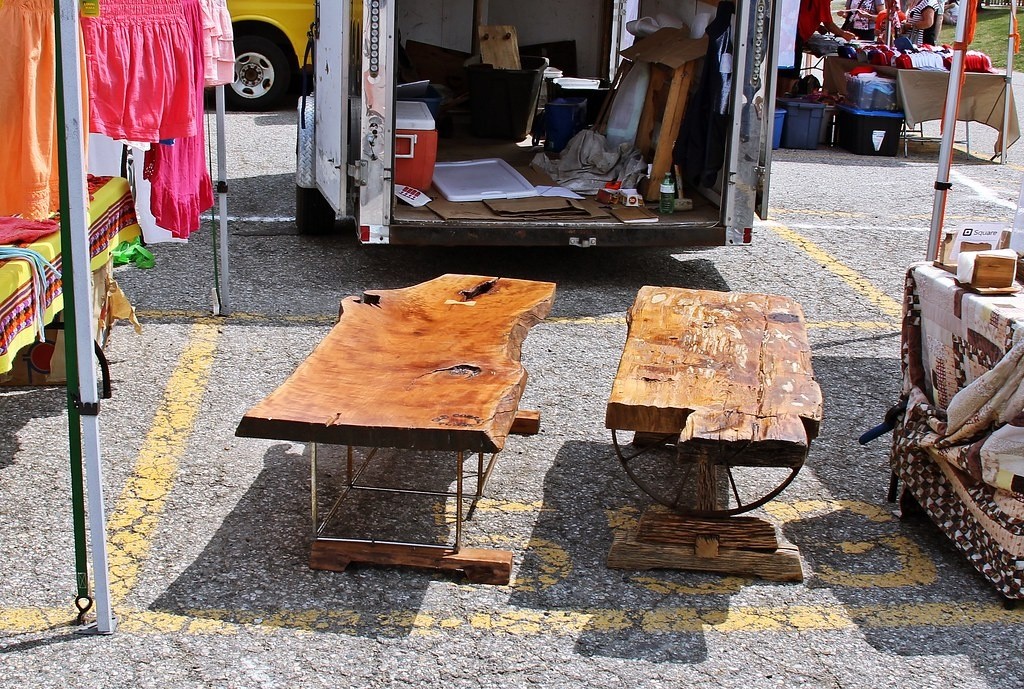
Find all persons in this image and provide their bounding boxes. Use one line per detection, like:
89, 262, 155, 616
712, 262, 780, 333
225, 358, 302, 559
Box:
836, 0, 960, 49
793, 0, 855, 77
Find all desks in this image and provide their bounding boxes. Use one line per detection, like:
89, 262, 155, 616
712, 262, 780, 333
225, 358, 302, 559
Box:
889, 262, 1024, 611
233, 274, 556, 587
608, 285, 823, 583
823, 55, 1021, 165
0, 177, 131, 398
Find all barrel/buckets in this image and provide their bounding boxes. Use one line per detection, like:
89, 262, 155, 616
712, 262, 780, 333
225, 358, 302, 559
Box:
545, 97, 587, 152
470, 55, 549, 141
397, 91, 443, 120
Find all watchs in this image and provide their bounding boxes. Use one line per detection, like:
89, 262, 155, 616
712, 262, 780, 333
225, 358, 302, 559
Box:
842, 13, 845, 17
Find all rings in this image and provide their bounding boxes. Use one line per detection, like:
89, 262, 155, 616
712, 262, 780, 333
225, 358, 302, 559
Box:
862, 13, 864, 15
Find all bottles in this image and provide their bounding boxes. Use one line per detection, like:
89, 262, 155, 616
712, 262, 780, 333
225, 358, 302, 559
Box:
659, 172, 675, 214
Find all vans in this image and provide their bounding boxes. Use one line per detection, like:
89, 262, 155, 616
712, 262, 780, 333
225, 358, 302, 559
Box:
203, 1, 317, 115
294, 0, 783, 252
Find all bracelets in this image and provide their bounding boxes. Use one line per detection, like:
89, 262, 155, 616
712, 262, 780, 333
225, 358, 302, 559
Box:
866, 14, 872, 20
910, 23, 913, 28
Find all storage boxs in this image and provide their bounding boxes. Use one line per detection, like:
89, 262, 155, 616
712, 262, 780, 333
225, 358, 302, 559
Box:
777, 99, 826, 149
833, 105, 904, 157
845, 71, 897, 112
469, 54, 550, 142
771, 107, 786, 150
546, 76, 617, 129
391, 99, 438, 192
547, 98, 587, 153
